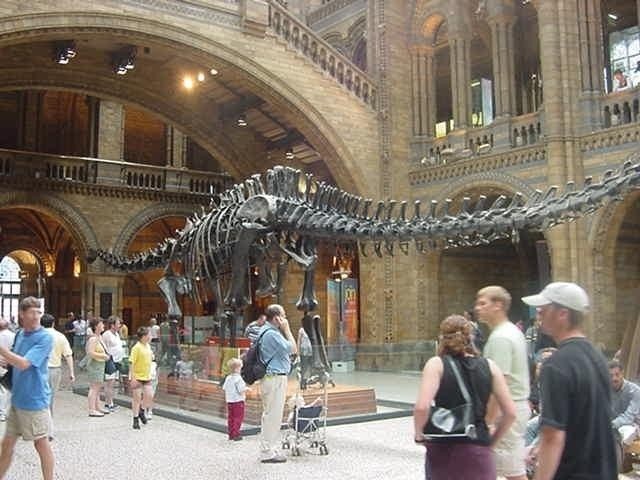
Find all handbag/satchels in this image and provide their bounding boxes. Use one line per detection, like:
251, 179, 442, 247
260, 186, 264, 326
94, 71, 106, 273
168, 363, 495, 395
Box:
422, 403, 477, 441
105, 353, 117, 375
1, 371, 12, 391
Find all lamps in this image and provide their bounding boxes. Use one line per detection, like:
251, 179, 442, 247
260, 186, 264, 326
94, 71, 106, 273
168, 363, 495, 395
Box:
54, 39, 294, 161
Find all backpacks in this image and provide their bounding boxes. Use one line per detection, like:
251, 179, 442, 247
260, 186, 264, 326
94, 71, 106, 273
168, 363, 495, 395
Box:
240, 328, 276, 385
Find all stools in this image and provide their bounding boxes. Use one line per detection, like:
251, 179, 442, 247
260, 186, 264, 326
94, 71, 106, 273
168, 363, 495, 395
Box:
620, 440, 640, 473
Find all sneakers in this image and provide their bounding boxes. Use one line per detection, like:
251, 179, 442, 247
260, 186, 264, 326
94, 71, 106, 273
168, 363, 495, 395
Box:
262, 455, 286, 462
229, 437, 242, 440
134, 408, 152, 429
89, 404, 120, 417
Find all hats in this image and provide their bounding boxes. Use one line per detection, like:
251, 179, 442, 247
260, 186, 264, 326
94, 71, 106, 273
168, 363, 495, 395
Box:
521, 282, 589, 313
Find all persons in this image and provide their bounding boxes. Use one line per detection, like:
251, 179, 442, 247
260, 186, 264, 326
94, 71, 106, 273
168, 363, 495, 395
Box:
256, 304, 299, 463
244, 313, 267, 347
297, 317, 314, 390
415, 281, 640, 478
0, 296, 162, 480
219, 357, 253, 441
612, 69, 633, 92
174, 349, 199, 412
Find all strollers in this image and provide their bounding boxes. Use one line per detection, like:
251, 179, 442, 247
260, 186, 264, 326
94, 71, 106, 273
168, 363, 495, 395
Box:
282, 372, 330, 456
